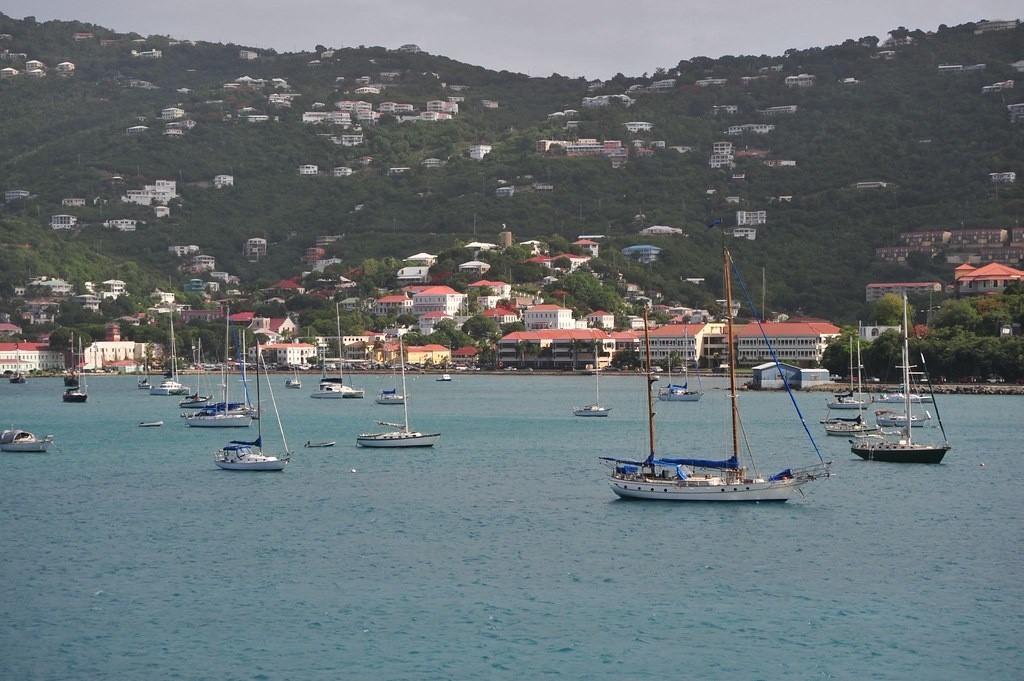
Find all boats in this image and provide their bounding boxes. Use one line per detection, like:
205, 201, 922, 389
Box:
436, 373, 452, 381
305, 441, 336, 448
210, 336, 295, 471
0, 426, 55, 452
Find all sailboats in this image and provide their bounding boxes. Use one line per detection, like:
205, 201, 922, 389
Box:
598, 242, 834, 505
572, 344, 613, 416
355, 333, 441, 450
821, 318, 934, 436
65, 331, 81, 388
62, 335, 89, 403
373, 358, 404, 405
658, 326, 704, 402
849, 290, 952, 464
284, 302, 366, 398
138, 301, 264, 428
8, 346, 26, 383
